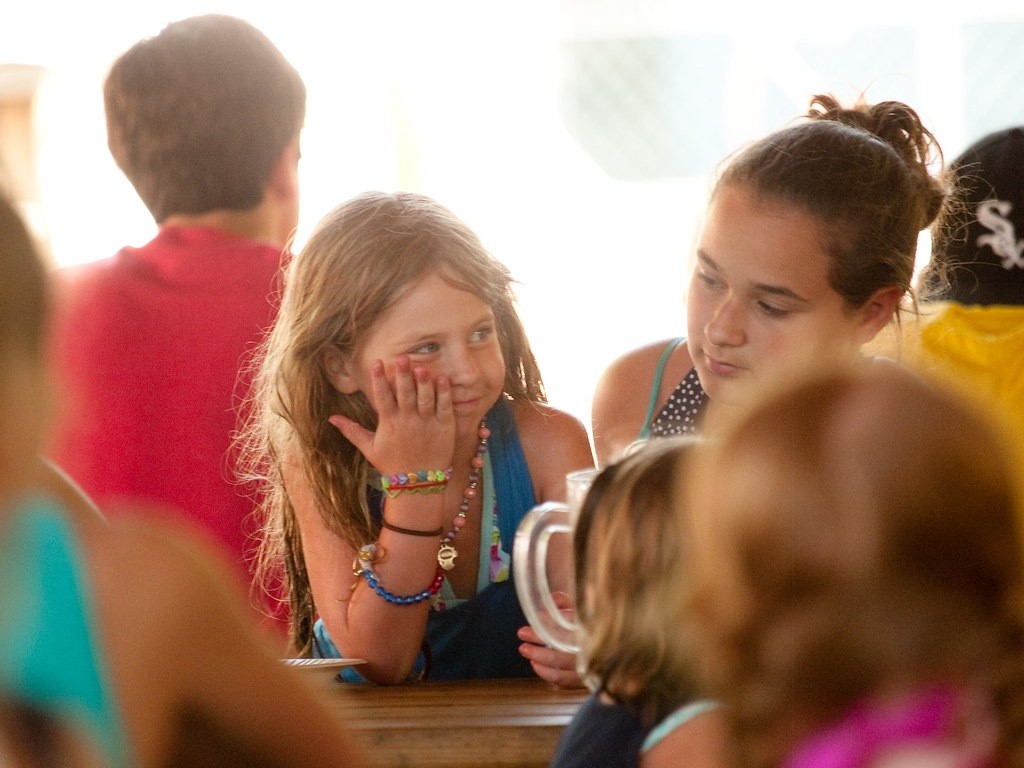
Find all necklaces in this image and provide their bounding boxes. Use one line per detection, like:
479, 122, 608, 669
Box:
444, 416, 490, 541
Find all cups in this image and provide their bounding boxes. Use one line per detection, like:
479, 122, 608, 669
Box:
512, 468, 604, 693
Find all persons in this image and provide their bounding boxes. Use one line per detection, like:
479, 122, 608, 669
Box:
34, 14, 320, 659
241, 189, 599, 691
0, 197, 363, 768
559, 99, 1024, 768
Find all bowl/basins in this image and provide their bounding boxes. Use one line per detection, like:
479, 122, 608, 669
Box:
280, 658, 366, 694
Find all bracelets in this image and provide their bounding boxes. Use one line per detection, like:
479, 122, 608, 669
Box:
382, 468, 453, 495
382, 521, 445, 537
355, 541, 446, 605
